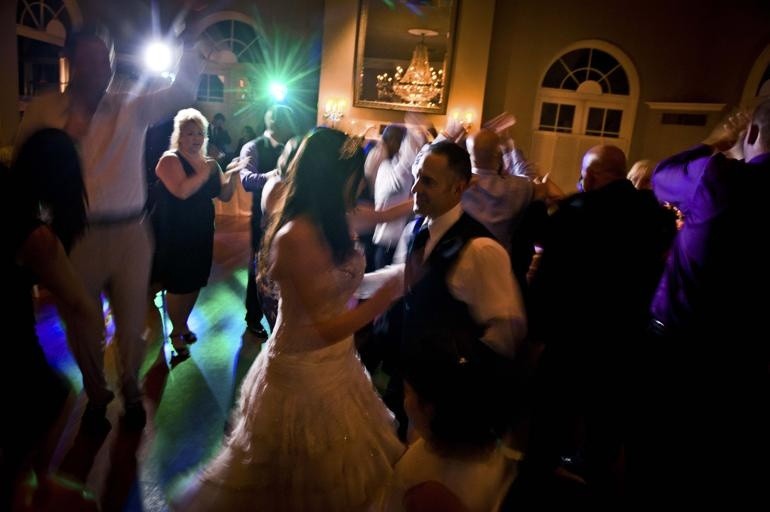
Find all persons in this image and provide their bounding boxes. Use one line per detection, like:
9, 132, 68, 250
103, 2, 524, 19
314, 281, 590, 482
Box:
168, 127, 405, 512
370, 341, 519, 512
626, 159, 659, 190
0, 125, 106, 472
372, 112, 436, 271
652, 97, 770, 338
509, 144, 677, 350
208, 113, 232, 155
362, 124, 406, 201
236, 126, 256, 154
239, 104, 300, 340
411, 118, 537, 247
153, 107, 252, 359
355, 139, 524, 354
18, 29, 221, 434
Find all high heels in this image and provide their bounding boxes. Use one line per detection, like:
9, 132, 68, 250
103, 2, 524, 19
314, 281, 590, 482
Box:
169, 334, 190, 358
183, 331, 197, 345
169, 351, 191, 370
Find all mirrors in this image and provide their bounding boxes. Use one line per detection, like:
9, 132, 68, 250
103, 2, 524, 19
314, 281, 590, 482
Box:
352, 1, 458, 116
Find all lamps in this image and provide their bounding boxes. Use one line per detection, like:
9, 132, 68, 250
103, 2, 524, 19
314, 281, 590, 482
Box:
322, 96, 348, 128
374, 26, 443, 106
454, 108, 477, 135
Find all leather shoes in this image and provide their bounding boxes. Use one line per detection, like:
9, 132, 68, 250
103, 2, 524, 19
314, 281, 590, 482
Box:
247, 321, 268, 339
